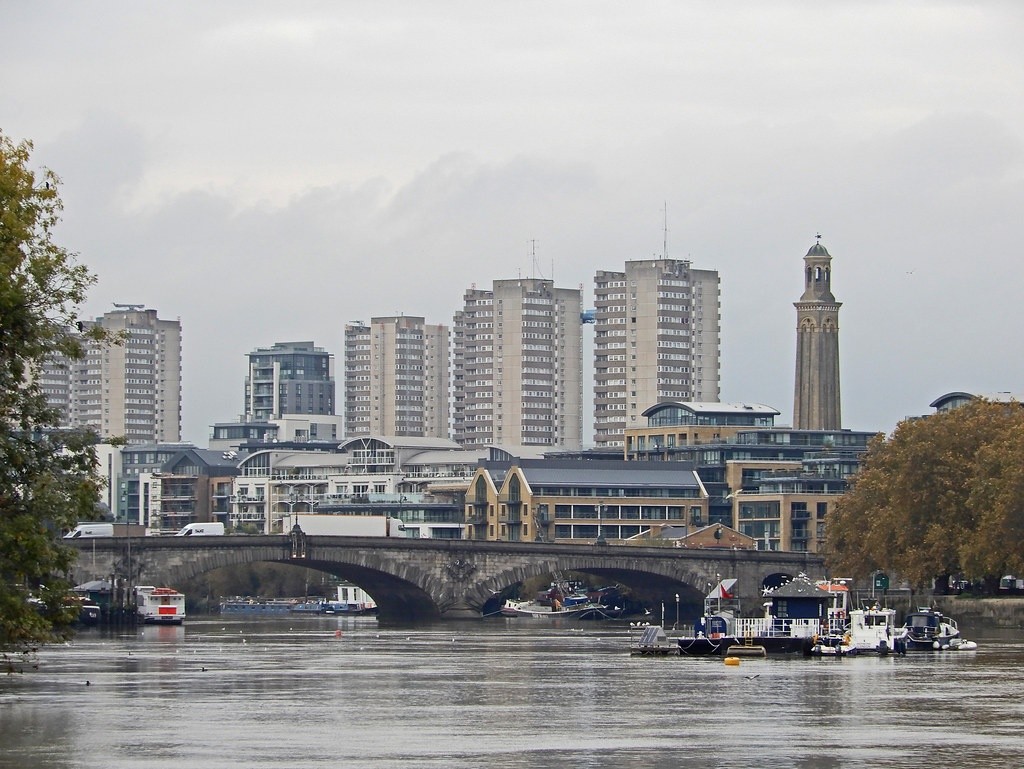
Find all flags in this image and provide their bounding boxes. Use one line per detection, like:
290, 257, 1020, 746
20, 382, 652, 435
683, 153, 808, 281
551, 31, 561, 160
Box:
721, 583, 735, 600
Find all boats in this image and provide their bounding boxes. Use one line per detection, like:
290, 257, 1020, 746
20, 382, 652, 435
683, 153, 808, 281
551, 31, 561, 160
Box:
14, 569, 982, 682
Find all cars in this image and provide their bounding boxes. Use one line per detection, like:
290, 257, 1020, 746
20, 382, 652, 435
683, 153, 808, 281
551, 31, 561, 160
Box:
950, 573, 1024, 593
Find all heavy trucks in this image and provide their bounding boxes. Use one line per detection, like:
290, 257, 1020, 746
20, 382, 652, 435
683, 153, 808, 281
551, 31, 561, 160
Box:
282, 514, 407, 538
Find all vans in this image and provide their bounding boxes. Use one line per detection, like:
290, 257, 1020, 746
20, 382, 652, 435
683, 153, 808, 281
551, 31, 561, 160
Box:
175, 522, 225, 537
63, 522, 114, 539
